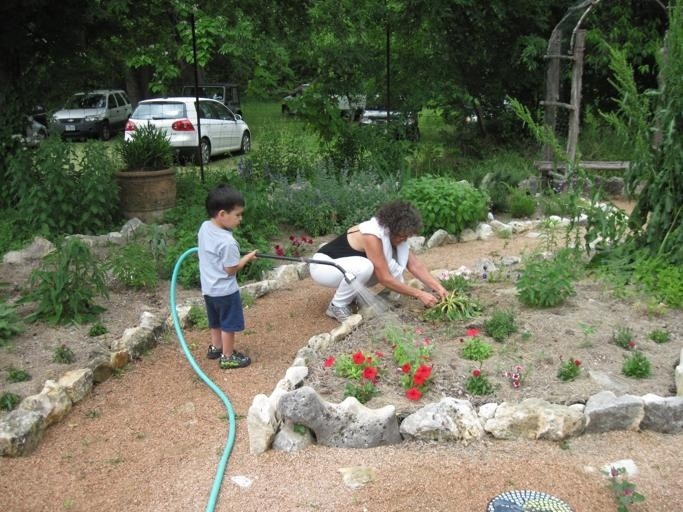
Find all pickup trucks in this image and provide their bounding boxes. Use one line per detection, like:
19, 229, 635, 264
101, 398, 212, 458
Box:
282, 83, 366, 122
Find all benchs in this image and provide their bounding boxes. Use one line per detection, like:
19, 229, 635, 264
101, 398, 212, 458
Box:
534, 160, 631, 190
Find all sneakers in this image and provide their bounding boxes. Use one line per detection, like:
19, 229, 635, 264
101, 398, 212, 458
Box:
325, 301, 353, 324
206, 343, 250, 369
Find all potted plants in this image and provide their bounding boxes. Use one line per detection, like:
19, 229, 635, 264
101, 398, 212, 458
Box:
114, 119, 177, 224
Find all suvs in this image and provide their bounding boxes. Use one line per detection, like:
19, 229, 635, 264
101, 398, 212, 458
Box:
51, 89, 131, 140
181, 82, 242, 119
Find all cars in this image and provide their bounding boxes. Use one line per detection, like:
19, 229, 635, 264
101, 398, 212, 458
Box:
11, 116, 47, 148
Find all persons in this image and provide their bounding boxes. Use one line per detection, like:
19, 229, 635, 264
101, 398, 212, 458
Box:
197, 184, 259, 369
309, 203, 449, 325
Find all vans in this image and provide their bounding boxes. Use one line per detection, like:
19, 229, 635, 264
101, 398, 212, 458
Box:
360, 92, 419, 140
124, 96, 250, 163
